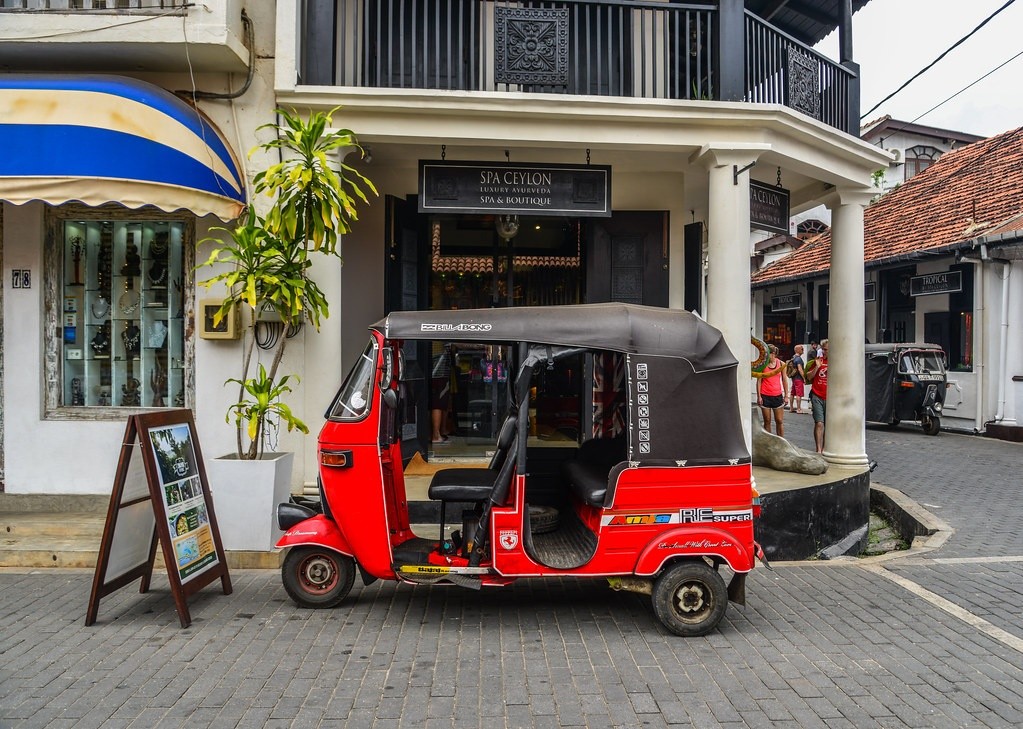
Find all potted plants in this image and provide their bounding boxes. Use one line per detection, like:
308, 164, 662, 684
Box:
188, 100, 380, 551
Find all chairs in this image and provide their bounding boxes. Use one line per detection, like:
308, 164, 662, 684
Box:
426, 409, 517, 555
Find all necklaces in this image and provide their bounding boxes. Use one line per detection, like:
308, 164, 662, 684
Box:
92, 304, 110, 319
119, 292, 141, 314
147, 269, 166, 284
89, 340, 108, 349
150, 238, 168, 255
122, 330, 139, 350
147, 326, 167, 340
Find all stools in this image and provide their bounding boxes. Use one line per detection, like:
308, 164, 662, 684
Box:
469, 399, 494, 434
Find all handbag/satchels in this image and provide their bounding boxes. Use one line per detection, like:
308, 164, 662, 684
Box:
804, 359, 816, 385
432, 353, 451, 385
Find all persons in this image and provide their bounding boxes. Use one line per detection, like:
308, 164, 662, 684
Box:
429, 341, 450, 443
756, 340, 828, 453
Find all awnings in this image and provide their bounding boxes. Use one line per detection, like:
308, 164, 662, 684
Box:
0, 73, 247, 222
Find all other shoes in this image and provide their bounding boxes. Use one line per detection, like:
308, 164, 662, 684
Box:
432, 438, 452, 443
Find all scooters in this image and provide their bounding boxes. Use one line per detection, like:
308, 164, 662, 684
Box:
430, 342, 507, 412
273, 301, 773, 636
513, 343, 582, 441
865, 343, 949, 435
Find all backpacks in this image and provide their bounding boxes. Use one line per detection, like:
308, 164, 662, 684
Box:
785, 356, 799, 378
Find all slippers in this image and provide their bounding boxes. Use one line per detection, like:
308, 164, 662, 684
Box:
797, 411, 808, 414
789, 410, 797, 413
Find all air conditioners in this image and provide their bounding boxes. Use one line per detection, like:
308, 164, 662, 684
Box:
888, 147, 906, 164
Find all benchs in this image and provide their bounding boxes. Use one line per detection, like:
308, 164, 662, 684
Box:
572, 437, 633, 512
